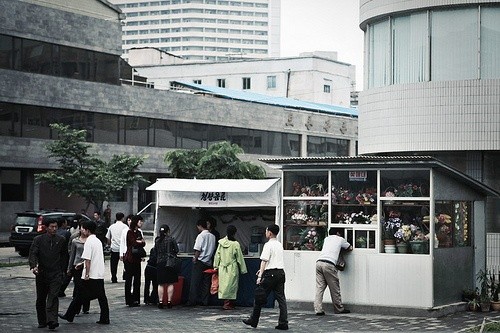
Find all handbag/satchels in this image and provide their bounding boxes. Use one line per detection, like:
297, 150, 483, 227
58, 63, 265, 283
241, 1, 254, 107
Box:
166, 241, 179, 269
210, 274, 219, 295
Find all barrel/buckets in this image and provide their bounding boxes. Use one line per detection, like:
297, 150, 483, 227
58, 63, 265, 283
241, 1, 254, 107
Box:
163, 276, 184, 306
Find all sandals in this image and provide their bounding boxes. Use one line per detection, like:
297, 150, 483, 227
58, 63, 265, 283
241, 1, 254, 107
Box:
159, 302, 164, 309
167, 301, 172, 308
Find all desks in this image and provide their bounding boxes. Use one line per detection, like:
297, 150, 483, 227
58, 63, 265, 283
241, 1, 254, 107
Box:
178, 253, 275, 308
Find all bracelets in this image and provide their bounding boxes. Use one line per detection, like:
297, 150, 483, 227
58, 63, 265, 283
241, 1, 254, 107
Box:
258, 277, 261, 278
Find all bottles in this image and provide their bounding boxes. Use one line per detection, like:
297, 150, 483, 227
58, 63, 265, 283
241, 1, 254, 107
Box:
177, 242, 183, 253
251, 226, 263, 243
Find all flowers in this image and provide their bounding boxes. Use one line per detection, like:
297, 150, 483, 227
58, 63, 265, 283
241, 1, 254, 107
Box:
286, 182, 452, 251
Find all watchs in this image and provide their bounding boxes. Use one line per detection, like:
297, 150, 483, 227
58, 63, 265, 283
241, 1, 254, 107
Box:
85, 275, 89, 277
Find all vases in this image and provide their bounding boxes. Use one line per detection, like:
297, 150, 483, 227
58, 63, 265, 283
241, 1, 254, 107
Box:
290, 235, 300, 242
384, 240, 429, 254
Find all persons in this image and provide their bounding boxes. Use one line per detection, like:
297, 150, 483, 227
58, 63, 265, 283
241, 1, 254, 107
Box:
314, 228, 353, 315
105, 212, 128, 284
67, 222, 90, 317
155, 224, 180, 309
184, 219, 216, 306
241, 223, 289, 330
93, 211, 107, 263
124, 215, 146, 307
120, 213, 144, 281
213, 225, 247, 310
143, 236, 161, 307
104, 204, 111, 227
56, 214, 87, 296
58, 221, 110, 324
193, 217, 220, 258
28, 219, 69, 330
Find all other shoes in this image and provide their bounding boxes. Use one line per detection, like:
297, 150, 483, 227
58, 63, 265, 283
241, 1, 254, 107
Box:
49, 323, 59, 330
75, 314, 79, 317
58, 313, 73, 322
336, 309, 349, 314
96, 320, 109, 325
242, 319, 257, 328
275, 324, 288, 330
38, 324, 46, 328
316, 312, 325, 316
223, 300, 232, 309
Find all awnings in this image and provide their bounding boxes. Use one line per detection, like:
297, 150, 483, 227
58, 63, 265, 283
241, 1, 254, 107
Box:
145, 178, 279, 192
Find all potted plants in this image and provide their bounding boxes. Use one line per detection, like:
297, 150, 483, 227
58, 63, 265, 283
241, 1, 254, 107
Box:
461, 269, 500, 312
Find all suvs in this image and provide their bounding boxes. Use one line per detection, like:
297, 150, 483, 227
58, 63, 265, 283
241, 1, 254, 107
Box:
10, 210, 111, 260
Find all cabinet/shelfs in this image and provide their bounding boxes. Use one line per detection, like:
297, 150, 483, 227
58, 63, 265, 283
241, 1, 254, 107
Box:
284, 201, 330, 244
